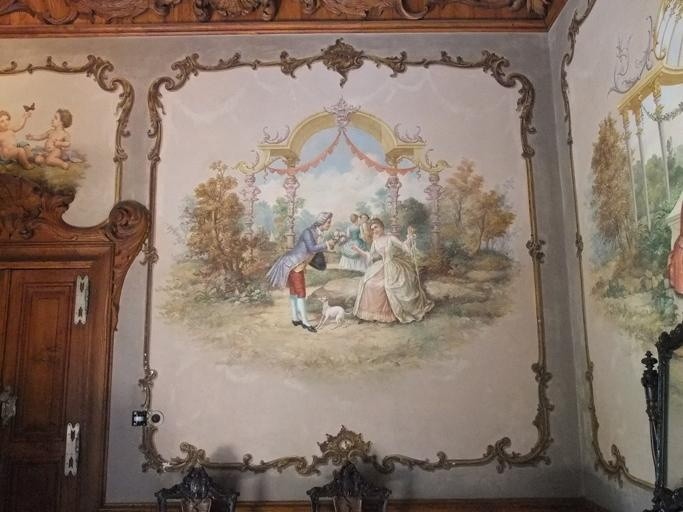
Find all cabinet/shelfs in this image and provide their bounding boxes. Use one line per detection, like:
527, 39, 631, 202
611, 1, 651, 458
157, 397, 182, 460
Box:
0, 167, 150, 511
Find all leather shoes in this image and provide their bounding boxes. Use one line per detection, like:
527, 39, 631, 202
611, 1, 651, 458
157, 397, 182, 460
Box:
292, 319, 302, 326
301, 323, 316, 332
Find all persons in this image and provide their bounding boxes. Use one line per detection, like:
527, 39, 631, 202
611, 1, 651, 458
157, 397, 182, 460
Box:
338, 213, 436, 324
266, 212, 337, 332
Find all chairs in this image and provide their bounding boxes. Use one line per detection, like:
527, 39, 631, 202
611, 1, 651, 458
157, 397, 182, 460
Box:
305, 460, 392, 512
153, 462, 241, 512
